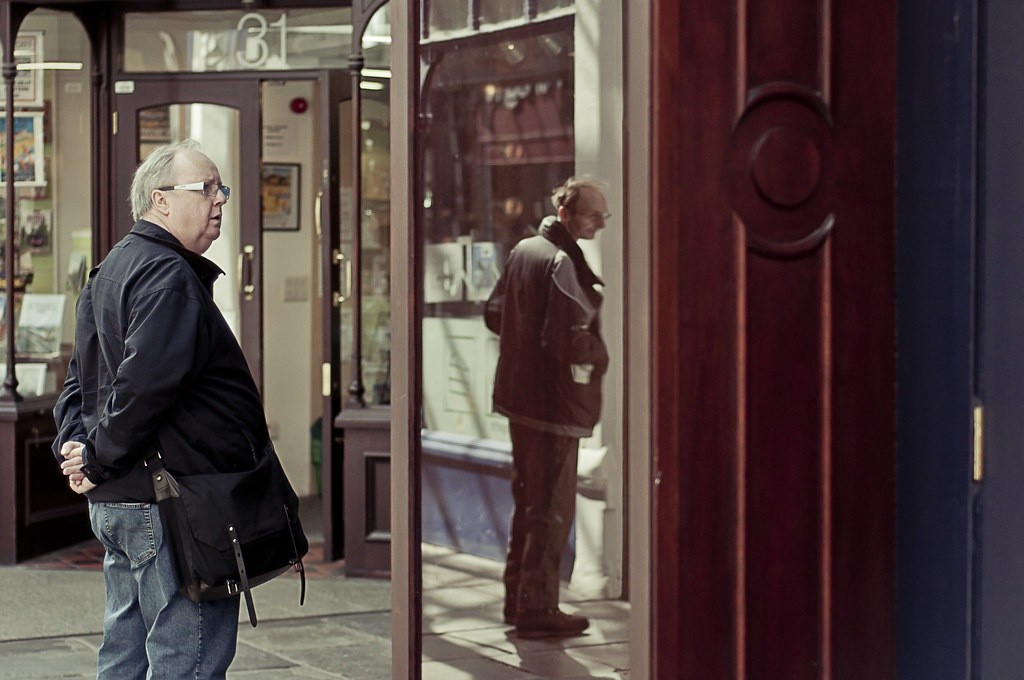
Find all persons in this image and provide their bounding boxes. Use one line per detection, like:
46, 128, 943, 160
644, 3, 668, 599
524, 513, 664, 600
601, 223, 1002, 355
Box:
484, 179, 608, 636
51, 140, 278, 680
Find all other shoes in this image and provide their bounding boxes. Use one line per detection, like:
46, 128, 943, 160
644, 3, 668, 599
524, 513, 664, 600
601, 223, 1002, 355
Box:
504, 608, 589, 640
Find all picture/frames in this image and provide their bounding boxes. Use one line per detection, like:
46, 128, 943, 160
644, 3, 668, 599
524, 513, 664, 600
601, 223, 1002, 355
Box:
263, 161, 302, 232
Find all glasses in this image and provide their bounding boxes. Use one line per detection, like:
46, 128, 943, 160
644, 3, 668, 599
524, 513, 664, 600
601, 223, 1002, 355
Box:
574, 209, 612, 222
151, 181, 231, 203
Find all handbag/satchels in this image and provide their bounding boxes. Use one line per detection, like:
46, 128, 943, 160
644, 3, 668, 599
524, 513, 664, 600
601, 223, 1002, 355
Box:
155, 451, 309, 628
